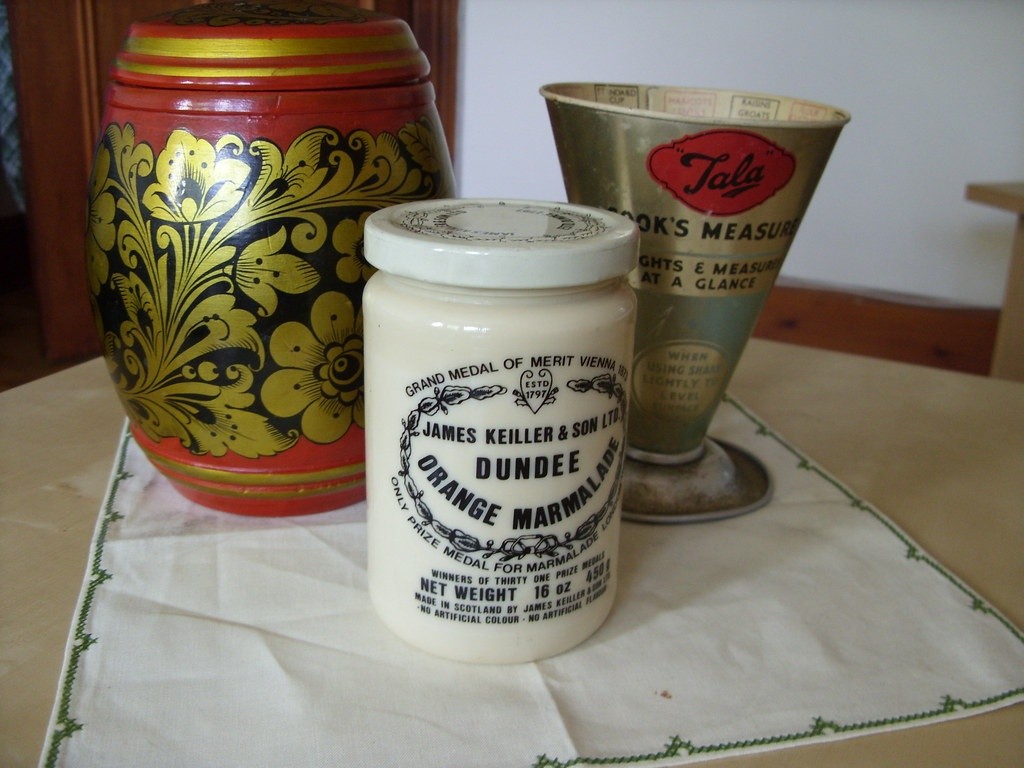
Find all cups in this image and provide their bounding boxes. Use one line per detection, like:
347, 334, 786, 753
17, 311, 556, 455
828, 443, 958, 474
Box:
539, 81, 850, 522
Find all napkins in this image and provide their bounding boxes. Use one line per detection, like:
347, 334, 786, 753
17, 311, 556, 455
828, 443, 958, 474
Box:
39, 393, 1024, 768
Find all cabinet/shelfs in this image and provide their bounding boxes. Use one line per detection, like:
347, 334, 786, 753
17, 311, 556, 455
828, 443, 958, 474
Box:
0, 0, 458, 393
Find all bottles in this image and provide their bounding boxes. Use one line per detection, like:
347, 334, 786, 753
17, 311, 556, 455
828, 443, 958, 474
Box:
85, 15, 461, 523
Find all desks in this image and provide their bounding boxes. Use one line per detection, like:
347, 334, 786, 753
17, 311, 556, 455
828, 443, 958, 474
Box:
965, 182, 1024, 382
